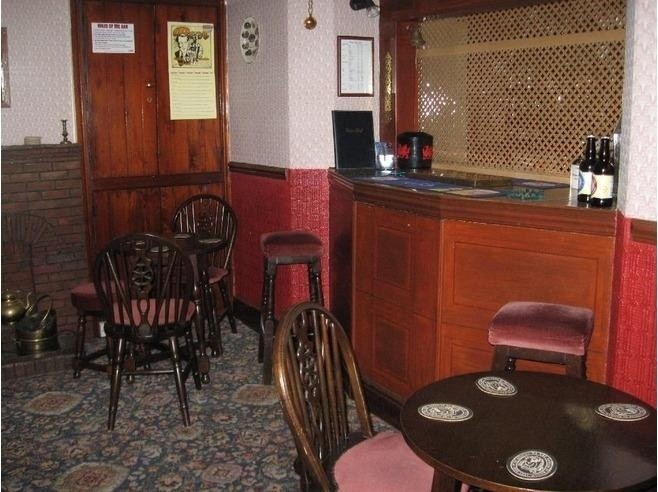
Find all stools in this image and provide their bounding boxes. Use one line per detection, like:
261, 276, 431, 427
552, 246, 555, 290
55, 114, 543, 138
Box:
487, 299, 594, 381
69, 279, 121, 379
257, 229, 326, 386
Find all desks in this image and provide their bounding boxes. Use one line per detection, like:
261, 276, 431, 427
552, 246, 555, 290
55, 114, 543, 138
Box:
398, 369, 656, 491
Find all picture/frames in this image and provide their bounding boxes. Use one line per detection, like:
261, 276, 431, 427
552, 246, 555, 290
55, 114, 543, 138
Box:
336, 35, 375, 98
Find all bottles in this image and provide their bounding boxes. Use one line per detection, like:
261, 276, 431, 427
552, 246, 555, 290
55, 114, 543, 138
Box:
377, 143, 394, 169
592, 135, 614, 207
578, 134, 596, 203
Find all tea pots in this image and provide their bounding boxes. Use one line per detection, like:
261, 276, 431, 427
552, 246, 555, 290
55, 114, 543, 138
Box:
14, 294, 60, 356
1, 286, 31, 327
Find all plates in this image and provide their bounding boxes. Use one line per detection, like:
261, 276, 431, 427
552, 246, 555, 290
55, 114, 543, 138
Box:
474, 373, 519, 399
417, 404, 474, 423
237, 17, 261, 63
504, 448, 560, 485
593, 401, 650, 422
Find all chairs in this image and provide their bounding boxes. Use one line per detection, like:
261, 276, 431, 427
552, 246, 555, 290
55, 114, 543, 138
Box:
91, 191, 240, 432
269, 302, 467, 490
